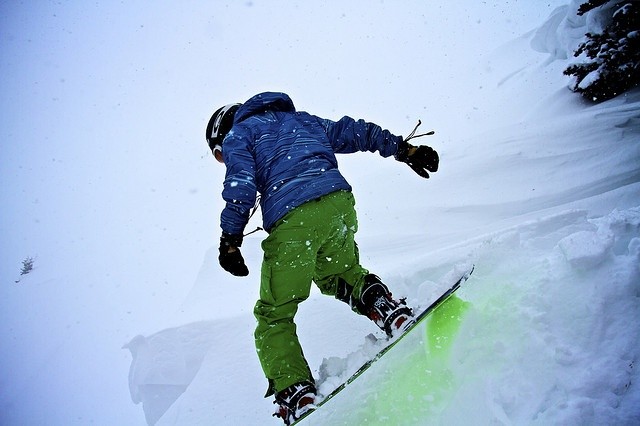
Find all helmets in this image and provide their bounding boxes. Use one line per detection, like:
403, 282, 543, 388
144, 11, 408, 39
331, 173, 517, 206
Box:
206, 103, 243, 162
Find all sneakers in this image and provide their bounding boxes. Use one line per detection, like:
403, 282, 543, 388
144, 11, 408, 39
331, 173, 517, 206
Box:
272, 381, 317, 425
369, 292, 412, 338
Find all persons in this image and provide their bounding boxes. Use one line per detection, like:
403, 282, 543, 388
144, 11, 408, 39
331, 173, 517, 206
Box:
205, 92, 439, 426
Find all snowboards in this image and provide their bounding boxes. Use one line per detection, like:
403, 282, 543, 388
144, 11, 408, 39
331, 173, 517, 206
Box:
287, 265, 475, 426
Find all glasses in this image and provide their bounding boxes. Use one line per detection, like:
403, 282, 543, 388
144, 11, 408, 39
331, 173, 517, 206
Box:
212, 145, 224, 164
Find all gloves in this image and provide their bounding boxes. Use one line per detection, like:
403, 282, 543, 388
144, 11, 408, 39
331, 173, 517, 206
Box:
395, 141, 439, 178
217, 231, 249, 276
